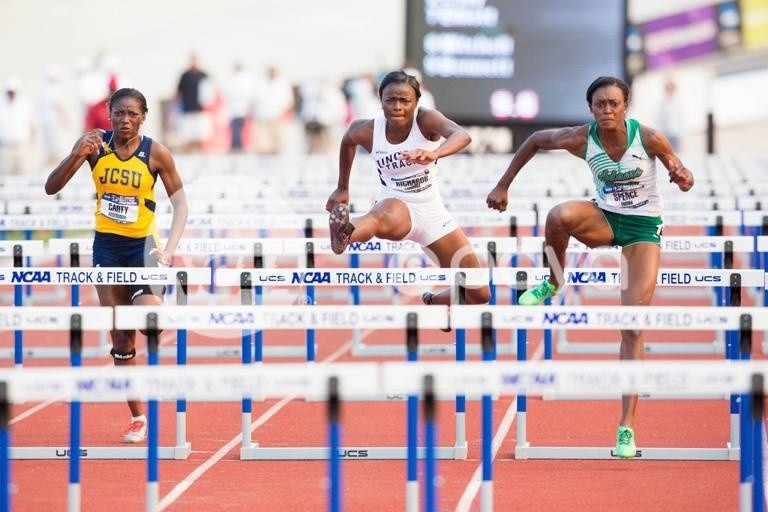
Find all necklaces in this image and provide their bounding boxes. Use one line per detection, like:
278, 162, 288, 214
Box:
101, 131, 139, 155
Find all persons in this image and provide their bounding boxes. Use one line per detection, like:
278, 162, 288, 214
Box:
45, 88, 188, 443
487, 77, 693, 457
326, 70, 491, 332
176, 53, 377, 152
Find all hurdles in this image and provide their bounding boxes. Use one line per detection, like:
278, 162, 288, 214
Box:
451, 305, 768, 512
491, 265, 766, 462
384, 359, 767, 512
113, 303, 450, 512
7, 361, 382, 512
213, 266, 491, 460
0, 266, 213, 463
0, 150, 768, 407
0, 305, 115, 512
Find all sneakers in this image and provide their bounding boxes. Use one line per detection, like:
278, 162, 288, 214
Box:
614, 424, 637, 459
328, 202, 355, 255
123, 414, 149, 444
422, 291, 453, 334
517, 279, 562, 306
